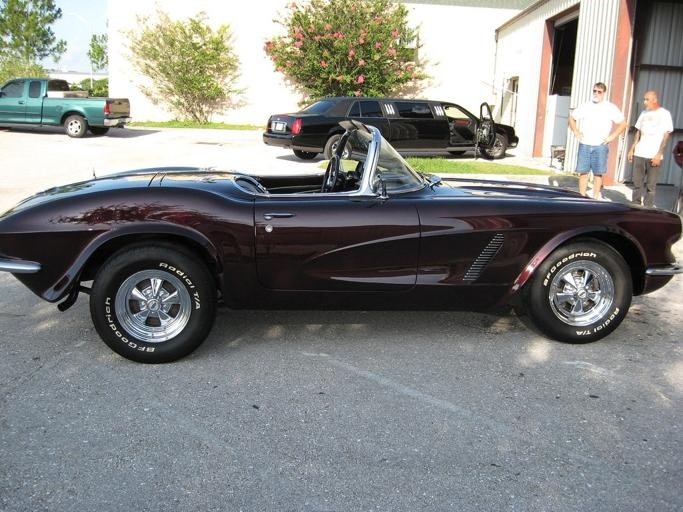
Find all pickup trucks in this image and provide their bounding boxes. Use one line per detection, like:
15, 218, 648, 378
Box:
1, 77, 132, 138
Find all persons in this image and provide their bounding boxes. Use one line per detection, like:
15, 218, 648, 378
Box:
568, 83, 626, 199
627, 90, 674, 207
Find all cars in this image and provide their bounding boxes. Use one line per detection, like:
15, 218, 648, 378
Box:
263, 95, 519, 161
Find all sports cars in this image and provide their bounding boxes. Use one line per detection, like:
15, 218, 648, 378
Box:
0, 113, 683, 364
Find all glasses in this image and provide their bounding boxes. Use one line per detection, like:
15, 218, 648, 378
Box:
593, 90, 602, 94
